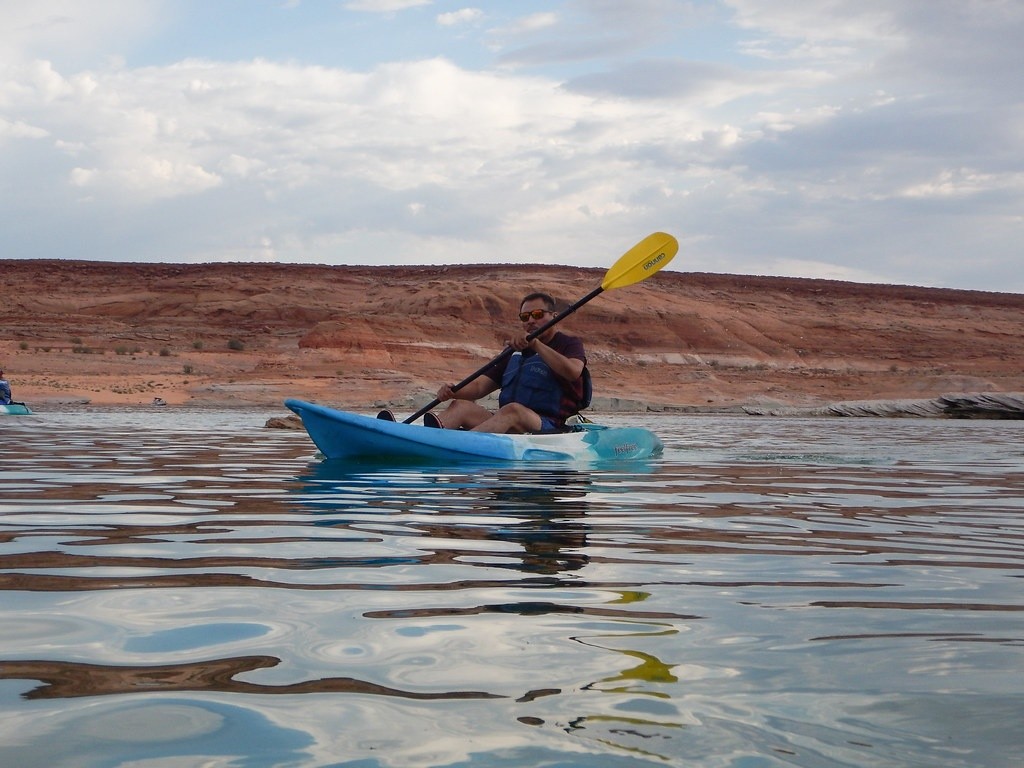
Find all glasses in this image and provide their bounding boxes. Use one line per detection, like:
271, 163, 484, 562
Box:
519, 308, 552, 322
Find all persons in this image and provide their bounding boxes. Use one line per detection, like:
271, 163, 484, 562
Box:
0, 368, 14, 404
376, 294, 588, 432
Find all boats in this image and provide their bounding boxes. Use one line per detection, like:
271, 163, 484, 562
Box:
284, 397, 664, 463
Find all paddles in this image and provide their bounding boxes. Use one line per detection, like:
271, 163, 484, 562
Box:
403, 232, 680, 424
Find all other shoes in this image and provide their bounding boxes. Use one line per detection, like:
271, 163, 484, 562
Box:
423, 412, 443, 429
377, 409, 396, 423
